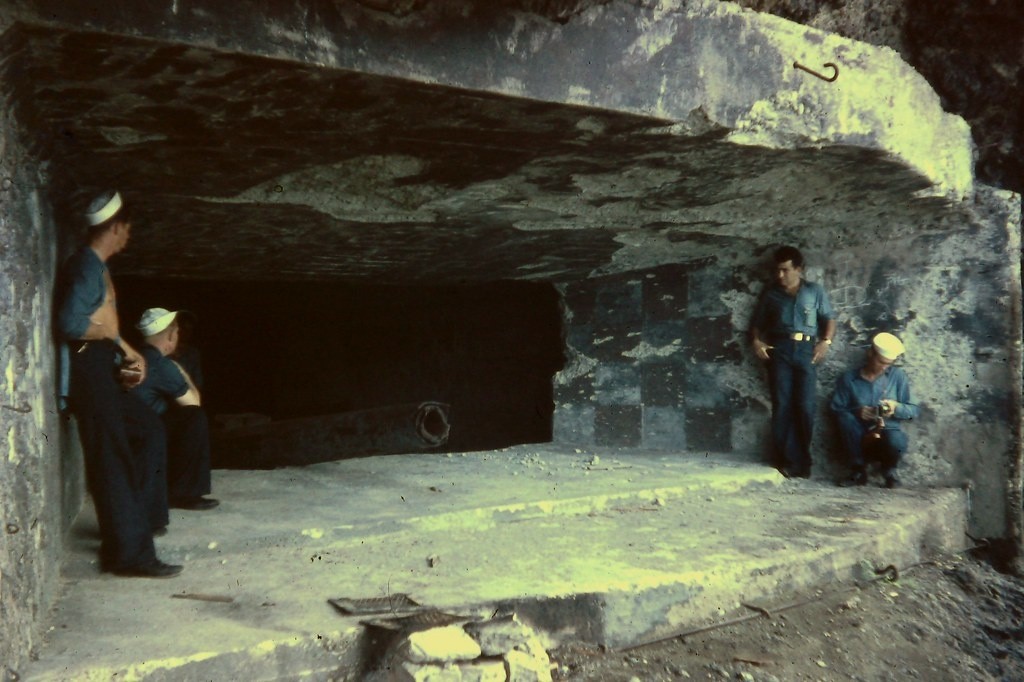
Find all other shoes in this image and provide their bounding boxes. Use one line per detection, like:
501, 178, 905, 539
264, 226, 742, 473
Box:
145, 562, 184, 578
839, 474, 867, 487
179, 493, 219, 509
152, 527, 169, 540
879, 474, 902, 489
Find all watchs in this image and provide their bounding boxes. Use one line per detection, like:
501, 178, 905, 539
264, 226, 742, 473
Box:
824, 339, 832, 346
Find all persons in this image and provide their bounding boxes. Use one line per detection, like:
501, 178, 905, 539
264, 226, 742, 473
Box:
747, 246, 834, 479
55, 187, 221, 577
829, 331, 920, 489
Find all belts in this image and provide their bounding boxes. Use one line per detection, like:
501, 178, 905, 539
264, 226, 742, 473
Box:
771, 332, 817, 342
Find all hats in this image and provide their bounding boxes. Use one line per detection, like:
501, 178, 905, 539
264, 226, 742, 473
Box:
83, 189, 122, 227
135, 308, 178, 337
872, 332, 906, 361
